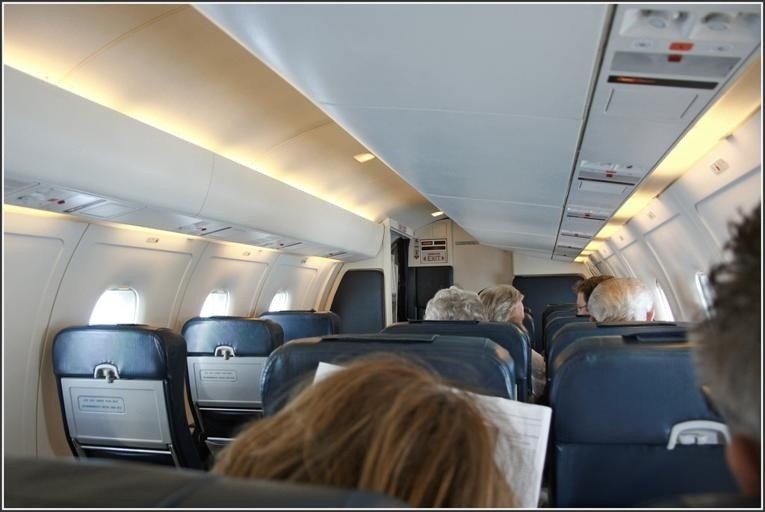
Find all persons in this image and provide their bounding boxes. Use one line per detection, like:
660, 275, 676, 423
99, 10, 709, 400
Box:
424, 274, 662, 397
684, 204, 761, 509
212, 352, 513, 508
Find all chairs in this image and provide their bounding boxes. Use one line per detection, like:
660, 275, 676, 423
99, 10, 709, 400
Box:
5, 304, 741, 509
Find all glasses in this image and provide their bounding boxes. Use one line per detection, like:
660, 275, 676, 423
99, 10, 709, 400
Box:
575, 303, 586, 312
